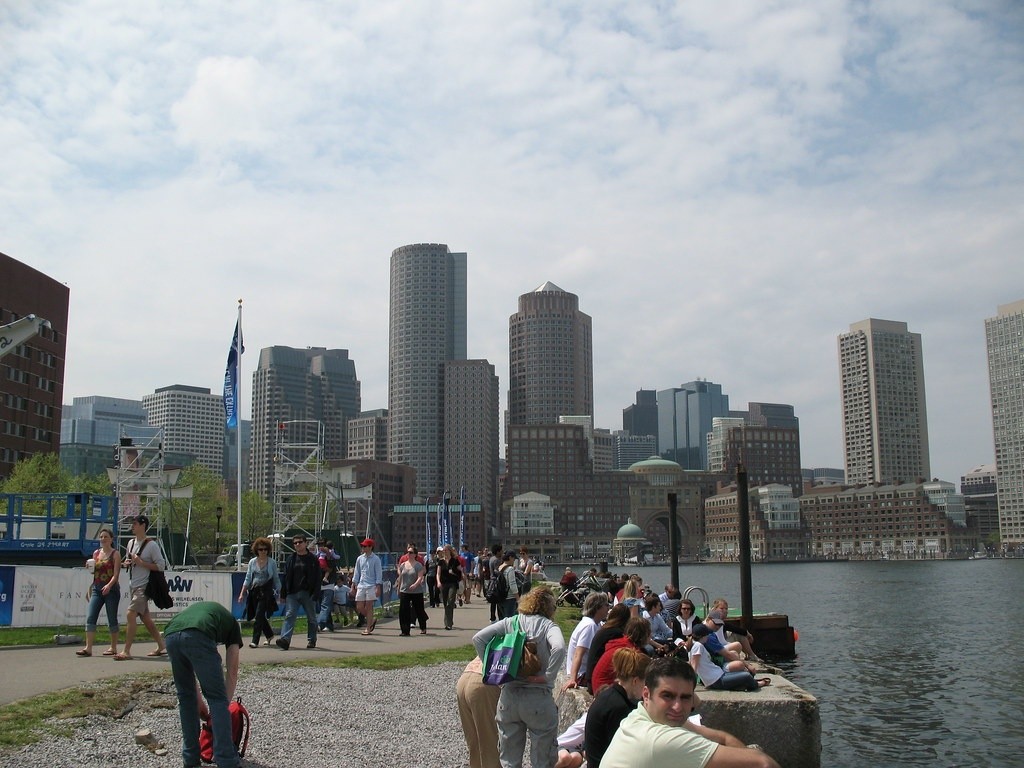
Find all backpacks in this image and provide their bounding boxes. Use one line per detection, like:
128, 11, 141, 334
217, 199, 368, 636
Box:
198, 697, 249, 763
486, 565, 514, 603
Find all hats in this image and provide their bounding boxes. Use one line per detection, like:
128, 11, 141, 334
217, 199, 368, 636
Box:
132, 514, 149, 524
360, 538, 376, 547
708, 610, 725, 625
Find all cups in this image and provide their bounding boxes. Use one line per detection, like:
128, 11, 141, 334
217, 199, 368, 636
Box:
88, 559, 95, 573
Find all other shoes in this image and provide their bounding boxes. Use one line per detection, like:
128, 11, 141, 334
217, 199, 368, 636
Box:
306, 640, 316, 648
317, 625, 321, 632
264, 636, 275, 645
323, 626, 332, 632
323, 578, 329, 583
275, 638, 289, 650
399, 620, 453, 637
249, 642, 257, 647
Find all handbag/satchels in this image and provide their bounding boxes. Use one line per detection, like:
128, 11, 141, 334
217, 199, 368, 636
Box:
482, 616, 527, 685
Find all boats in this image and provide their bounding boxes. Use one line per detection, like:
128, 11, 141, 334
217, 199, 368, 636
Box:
684, 585, 796, 657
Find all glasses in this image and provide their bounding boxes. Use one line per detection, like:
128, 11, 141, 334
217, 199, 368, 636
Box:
258, 548, 269, 552
406, 551, 415, 555
680, 607, 691, 611
294, 540, 305, 545
361, 545, 369, 548
709, 618, 724, 627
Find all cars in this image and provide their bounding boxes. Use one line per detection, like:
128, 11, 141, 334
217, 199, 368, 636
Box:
211, 542, 251, 567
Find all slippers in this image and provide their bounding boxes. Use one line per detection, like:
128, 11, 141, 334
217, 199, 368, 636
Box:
113, 654, 133, 660
334, 614, 377, 635
756, 678, 771, 686
103, 650, 118, 655
147, 651, 168, 656
76, 650, 93, 656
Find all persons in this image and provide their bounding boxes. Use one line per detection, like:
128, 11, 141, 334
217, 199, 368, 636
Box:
599, 657, 780, 768
113, 515, 168, 661
239, 534, 382, 650
76, 528, 121, 656
663, 589, 683, 621
560, 593, 771, 693
585, 648, 652, 768
397, 541, 545, 637
561, 566, 648, 606
472, 585, 567, 768
658, 584, 674, 606
554, 711, 588, 768
457, 655, 503, 768
164, 601, 244, 768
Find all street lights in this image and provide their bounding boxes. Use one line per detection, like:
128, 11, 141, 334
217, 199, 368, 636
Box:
215, 506, 222, 554
444, 489, 453, 531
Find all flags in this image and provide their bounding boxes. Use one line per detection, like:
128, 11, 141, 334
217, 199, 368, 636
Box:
223, 317, 245, 427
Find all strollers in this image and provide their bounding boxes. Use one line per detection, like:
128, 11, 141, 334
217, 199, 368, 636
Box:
554, 574, 598, 608
